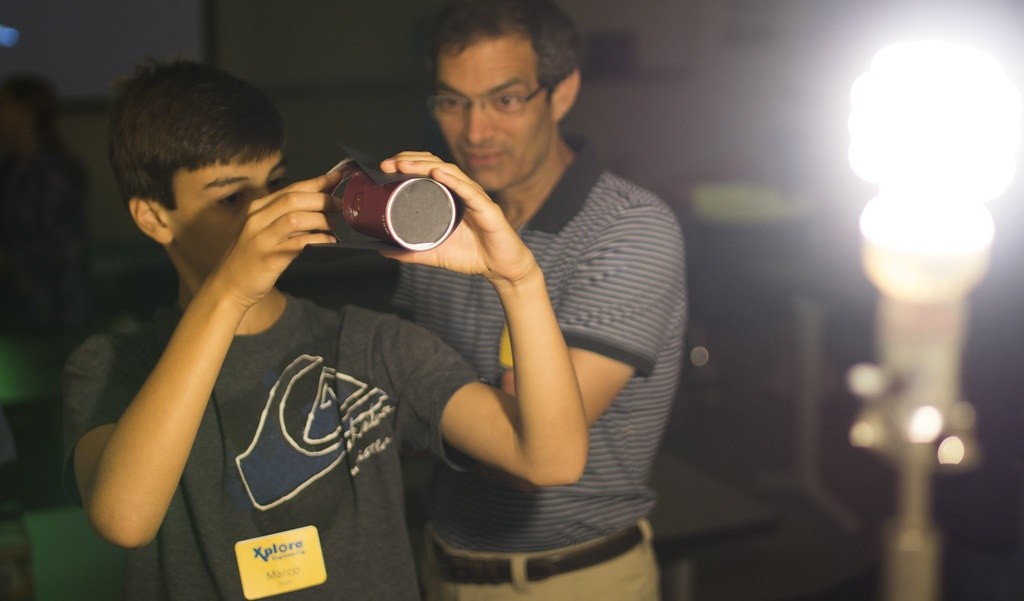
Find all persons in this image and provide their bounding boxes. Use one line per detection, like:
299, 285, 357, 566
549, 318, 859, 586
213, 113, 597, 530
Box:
63, 62, 590, 601
369, 1, 686, 601
0, 72, 114, 520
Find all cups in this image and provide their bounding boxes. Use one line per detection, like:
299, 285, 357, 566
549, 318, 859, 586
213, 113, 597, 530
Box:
325, 155, 456, 251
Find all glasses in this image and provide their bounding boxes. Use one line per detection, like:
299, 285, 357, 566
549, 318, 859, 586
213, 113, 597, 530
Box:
425, 85, 546, 116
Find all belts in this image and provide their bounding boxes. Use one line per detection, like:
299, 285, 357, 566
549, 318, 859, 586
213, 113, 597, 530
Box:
416, 524, 641, 581
